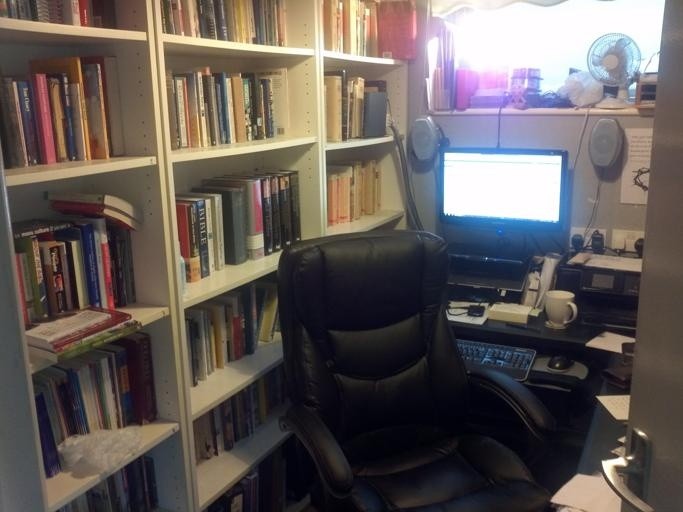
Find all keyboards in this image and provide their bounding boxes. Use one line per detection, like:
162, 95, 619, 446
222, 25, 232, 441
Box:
456, 338, 537, 382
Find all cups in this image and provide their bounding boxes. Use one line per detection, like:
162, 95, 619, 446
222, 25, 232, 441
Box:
545, 290, 578, 327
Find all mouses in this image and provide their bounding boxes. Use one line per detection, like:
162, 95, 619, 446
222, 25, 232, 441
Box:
547, 354, 575, 372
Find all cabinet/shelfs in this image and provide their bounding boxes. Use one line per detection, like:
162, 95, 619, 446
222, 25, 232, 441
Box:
0, 0, 412, 512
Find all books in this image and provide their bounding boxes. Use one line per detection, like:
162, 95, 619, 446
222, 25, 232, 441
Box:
161, 0, 287, 47
0, 0, 116, 29
326, 159, 382, 226
194, 361, 284, 466
59, 455, 159, 512
175, 167, 301, 283
206, 433, 309, 512
323, 0, 417, 59
32, 331, 157, 477
323, 70, 387, 142
0, 56, 124, 168
183, 282, 280, 388
166, 66, 288, 150
11, 191, 143, 363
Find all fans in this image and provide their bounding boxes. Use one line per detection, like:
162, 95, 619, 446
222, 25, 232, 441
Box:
586, 34, 642, 109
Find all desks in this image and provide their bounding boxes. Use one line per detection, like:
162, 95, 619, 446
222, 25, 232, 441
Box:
443, 288, 637, 395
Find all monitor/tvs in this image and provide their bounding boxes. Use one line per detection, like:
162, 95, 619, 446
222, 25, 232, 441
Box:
437, 146, 568, 266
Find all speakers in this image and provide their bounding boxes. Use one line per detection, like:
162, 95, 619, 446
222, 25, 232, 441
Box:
586, 118, 624, 168
411, 114, 438, 162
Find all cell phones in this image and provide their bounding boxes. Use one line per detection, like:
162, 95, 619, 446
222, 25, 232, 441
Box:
468, 304, 486, 317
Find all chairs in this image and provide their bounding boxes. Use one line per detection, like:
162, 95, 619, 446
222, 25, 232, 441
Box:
278, 227, 554, 510
